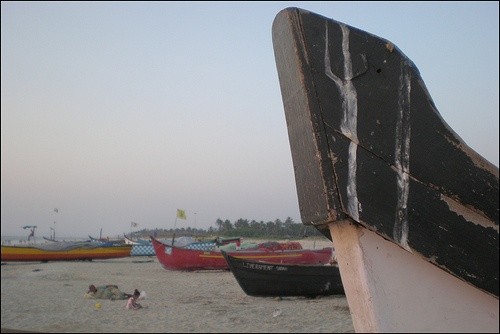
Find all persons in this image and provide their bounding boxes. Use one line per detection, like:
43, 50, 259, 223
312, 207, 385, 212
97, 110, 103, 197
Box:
87, 284, 97, 294
126, 289, 141, 309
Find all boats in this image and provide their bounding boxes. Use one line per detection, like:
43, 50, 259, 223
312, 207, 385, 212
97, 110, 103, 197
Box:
1, 236, 240, 261
268, 6, 500, 331
149, 235, 335, 272
219, 245, 346, 297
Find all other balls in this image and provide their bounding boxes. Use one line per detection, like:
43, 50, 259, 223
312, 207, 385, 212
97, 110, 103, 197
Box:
95, 303, 102, 307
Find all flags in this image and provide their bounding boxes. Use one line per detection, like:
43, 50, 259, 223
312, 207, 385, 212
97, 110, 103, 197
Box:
176, 209, 187, 220
131, 221, 137, 227
